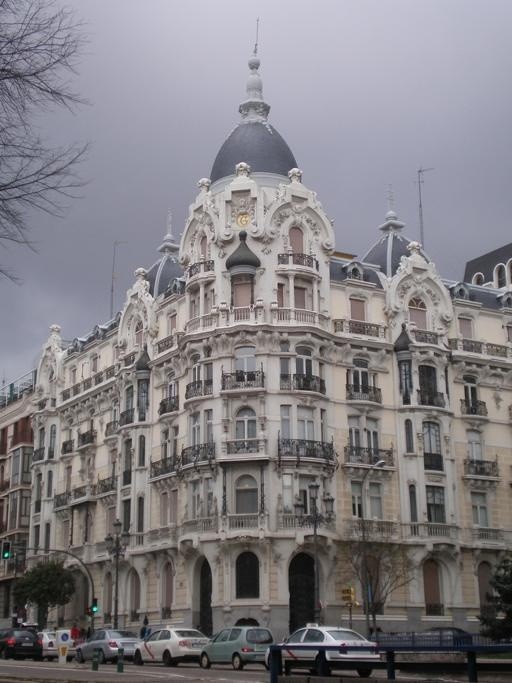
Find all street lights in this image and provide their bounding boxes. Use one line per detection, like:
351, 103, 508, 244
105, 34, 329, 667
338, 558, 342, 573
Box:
293, 483, 335, 626
104, 518, 130, 628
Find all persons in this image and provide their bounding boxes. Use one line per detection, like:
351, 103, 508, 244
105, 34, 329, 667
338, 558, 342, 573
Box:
71, 623, 91, 640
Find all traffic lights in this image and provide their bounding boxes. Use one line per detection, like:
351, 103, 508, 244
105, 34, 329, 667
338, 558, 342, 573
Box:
2, 542, 10, 558
91, 598, 97, 611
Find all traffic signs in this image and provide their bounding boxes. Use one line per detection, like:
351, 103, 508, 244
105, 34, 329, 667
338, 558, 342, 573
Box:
342, 588, 353, 594
347, 602, 360, 608
342, 596, 352, 601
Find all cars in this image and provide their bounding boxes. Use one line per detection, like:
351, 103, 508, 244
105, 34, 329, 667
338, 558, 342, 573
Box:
75, 628, 143, 663
200, 625, 276, 669
36, 630, 77, 661
132, 627, 212, 666
264, 622, 381, 677
0, 627, 42, 660
